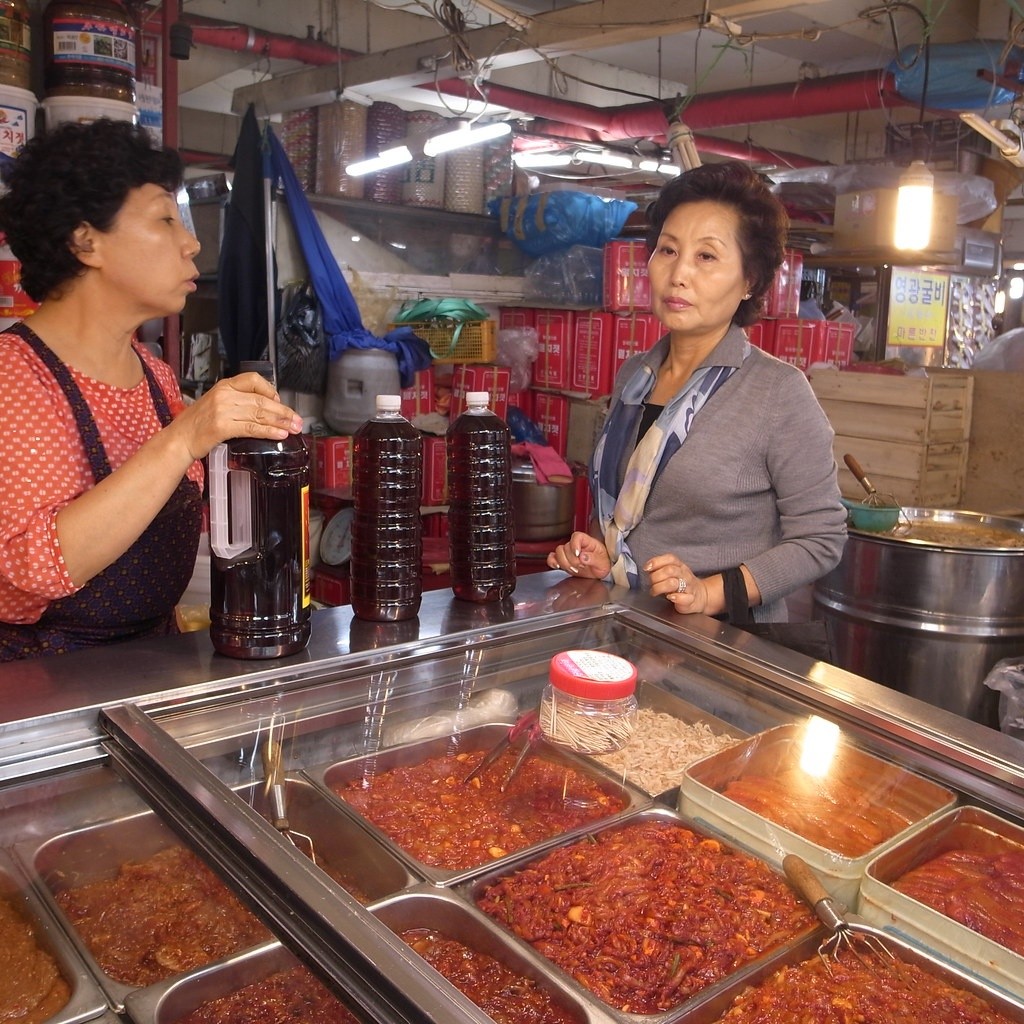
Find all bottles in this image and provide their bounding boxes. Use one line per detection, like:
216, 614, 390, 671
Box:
445, 391, 518, 602
348, 394, 422, 622
206, 362, 312, 660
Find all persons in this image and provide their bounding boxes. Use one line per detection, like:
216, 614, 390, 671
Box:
0, 117, 311, 665
545, 162, 850, 626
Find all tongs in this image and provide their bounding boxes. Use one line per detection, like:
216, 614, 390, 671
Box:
460, 703, 542, 793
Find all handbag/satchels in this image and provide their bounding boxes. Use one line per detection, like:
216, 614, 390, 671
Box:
721, 566, 842, 668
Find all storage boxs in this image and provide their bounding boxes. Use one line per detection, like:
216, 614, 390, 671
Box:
1, 241, 1023, 1022
831, 186, 958, 254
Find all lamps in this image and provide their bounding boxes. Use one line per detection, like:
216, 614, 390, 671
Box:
508, 147, 634, 170
638, 153, 681, 177
343, 121, 513, 178
467, 0, 532, 32
959, 106, 1024, 171
892, 132, 936, 252
662, 103, 701, 175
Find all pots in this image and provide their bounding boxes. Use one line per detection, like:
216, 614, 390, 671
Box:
510, 456, 579, 542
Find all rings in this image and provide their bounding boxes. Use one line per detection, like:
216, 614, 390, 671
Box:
675, 577, 687, 594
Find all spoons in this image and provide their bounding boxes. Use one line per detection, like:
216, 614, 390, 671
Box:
839, 496, 903, 533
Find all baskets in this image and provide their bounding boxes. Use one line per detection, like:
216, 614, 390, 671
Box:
386, 319, 498, 365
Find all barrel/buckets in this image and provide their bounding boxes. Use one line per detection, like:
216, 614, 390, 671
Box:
40, 95, 142, 135
323, 347, 401, 433
805, 506, 1022, 730
0, 229, 45, 337
40, 1, 140, 104
0, 83, 39, 199
1, 0, 38, 92
